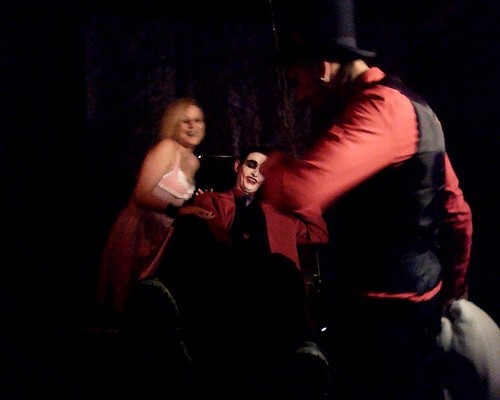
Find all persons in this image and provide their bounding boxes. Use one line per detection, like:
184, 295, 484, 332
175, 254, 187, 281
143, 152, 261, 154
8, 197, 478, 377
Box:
96, 97, 214, 312
261, 29, 492, 399
168, 146, 331, 366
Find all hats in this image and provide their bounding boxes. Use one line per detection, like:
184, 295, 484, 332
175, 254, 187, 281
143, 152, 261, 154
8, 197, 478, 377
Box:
248, 2, 378, 64
